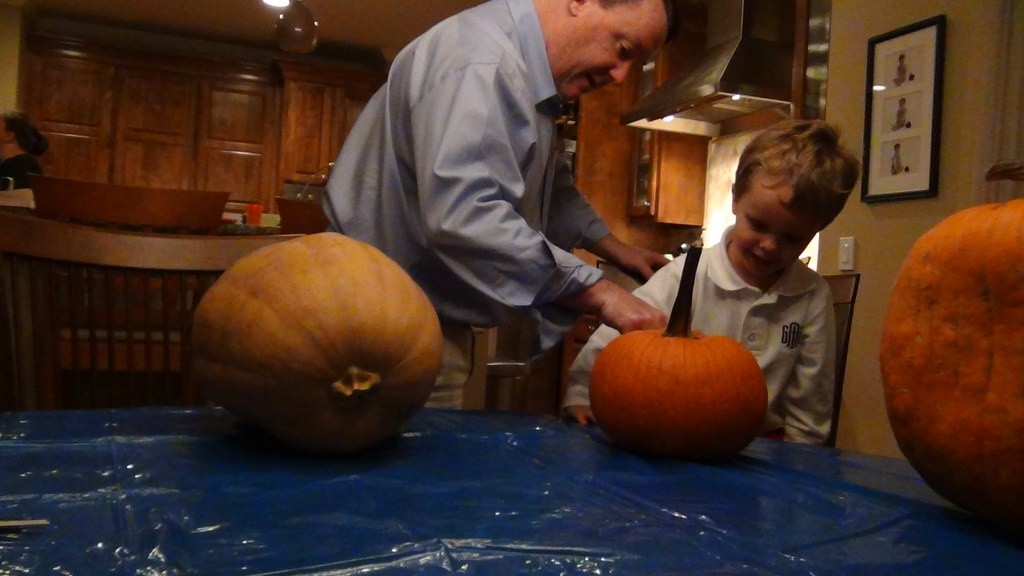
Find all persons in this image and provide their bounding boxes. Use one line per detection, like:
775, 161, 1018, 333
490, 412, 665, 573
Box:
318, 0, 677, 410
0, 112, 49, 191
562, 119, 862, 445
890, 53, 909, 175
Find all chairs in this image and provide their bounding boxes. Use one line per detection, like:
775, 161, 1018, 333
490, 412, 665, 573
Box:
594, 261, 860, 448
0, 211, 307, 413
23, 175, 231, 407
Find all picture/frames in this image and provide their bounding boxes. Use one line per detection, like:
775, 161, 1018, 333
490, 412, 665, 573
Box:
861, 14, 949, 204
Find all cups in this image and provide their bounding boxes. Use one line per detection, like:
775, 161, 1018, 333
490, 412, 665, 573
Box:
260, 214, 280, 226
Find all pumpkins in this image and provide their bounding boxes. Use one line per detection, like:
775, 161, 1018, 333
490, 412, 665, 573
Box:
881, 160, 1024, 523
185, 231, 442, 456
588, 241, 769, 463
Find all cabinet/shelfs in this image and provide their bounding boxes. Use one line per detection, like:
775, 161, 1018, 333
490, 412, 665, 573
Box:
627, 39, 707, 228
14, 9, 280, 214
273, 53, 388, 186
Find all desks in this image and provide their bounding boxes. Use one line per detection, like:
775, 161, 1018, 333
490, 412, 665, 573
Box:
0, 407, 1023, 576
